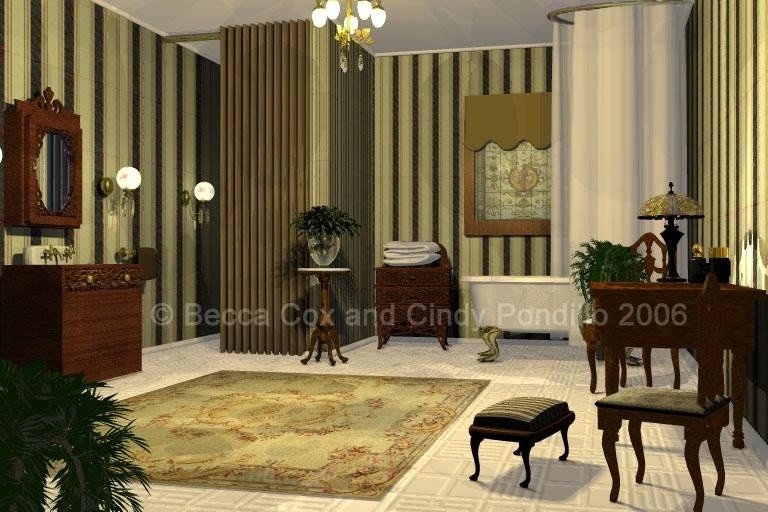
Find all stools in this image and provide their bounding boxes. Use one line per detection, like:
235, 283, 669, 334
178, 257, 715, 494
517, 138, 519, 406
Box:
467, 393, 574, 495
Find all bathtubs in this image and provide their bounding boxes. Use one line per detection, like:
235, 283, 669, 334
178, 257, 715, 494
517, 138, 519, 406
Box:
459, 275, 643, 367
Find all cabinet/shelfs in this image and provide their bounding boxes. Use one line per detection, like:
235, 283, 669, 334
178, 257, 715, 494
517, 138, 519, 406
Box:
1, 262, 154, 388
0, 86, 85, 229
370, 237, 453, 350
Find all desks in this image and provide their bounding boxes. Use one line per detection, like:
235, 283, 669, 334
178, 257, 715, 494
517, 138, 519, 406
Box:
293, 263, 353, 367
585, 278, 759, 455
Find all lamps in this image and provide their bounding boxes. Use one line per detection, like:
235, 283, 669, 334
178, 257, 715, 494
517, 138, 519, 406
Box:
179, 181, 216, 228
638, 184, 707, 283
311, 1, 387, 74
100, 164, 144, 216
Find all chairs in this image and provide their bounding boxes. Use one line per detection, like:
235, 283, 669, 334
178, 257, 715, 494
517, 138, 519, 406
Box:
590, 271, 732, 512
585, 228, 686, 396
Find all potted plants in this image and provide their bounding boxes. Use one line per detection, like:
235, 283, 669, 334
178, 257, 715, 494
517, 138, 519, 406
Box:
290, 203, 362, 266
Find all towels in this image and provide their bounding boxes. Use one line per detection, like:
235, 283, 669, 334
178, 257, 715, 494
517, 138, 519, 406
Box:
135, 248, 158, 281
382, 251, 441, 267
382, 240, 442, 254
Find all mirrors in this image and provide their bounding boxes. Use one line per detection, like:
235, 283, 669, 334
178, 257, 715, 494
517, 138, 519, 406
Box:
34, 127, 75, 214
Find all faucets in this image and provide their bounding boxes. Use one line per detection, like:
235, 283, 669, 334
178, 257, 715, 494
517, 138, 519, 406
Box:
52, 247, 65, 261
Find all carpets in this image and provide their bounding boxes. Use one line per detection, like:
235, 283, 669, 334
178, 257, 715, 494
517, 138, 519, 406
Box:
42, 364, 492, 504
